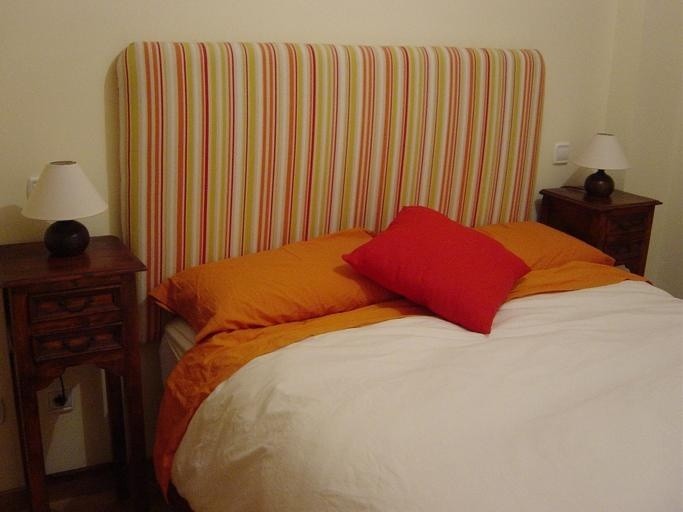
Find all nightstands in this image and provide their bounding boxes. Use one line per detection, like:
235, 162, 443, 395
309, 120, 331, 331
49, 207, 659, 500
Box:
539, 186, 663, 277
0, 235, 149, 512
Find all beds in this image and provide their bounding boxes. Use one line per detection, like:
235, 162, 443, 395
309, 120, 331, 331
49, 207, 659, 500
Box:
117, 40, 683, 512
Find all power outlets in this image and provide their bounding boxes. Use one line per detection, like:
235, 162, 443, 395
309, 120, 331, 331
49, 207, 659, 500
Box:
48, 389, 73, 416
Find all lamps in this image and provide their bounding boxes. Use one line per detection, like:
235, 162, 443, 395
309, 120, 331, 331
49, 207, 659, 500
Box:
20, 161, 109, 257
575, 133, 630, 201
